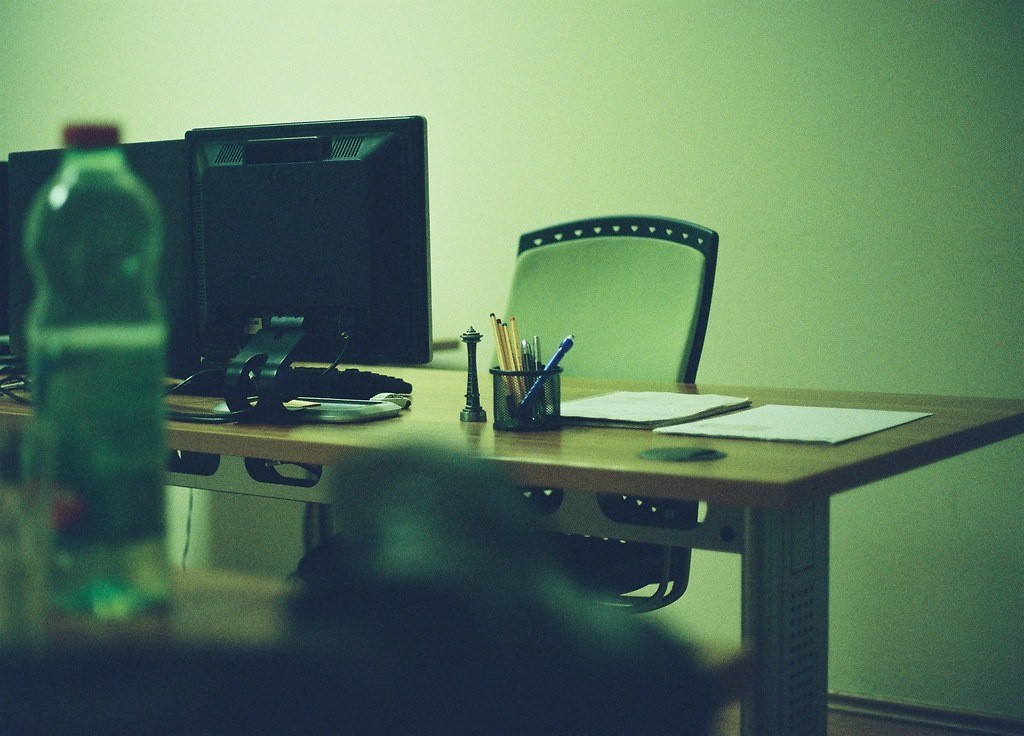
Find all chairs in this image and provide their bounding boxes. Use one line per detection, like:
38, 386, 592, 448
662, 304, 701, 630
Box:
497, 211, 719, 614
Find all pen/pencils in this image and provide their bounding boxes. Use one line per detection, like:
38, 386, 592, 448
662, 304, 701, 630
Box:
519, 334, 576, 413
489, 311, 543, 427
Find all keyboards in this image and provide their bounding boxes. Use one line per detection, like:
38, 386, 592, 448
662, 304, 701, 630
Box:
184, 361, 412, 401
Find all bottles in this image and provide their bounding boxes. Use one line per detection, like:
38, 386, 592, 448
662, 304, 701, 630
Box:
17, 122, 174, 621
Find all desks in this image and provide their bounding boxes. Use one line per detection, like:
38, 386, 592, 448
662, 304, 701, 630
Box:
0, 363, 1024, 736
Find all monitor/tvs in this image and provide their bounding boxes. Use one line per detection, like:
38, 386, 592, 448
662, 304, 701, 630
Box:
7, 140, 198, 377
189, 115, 433, 422
